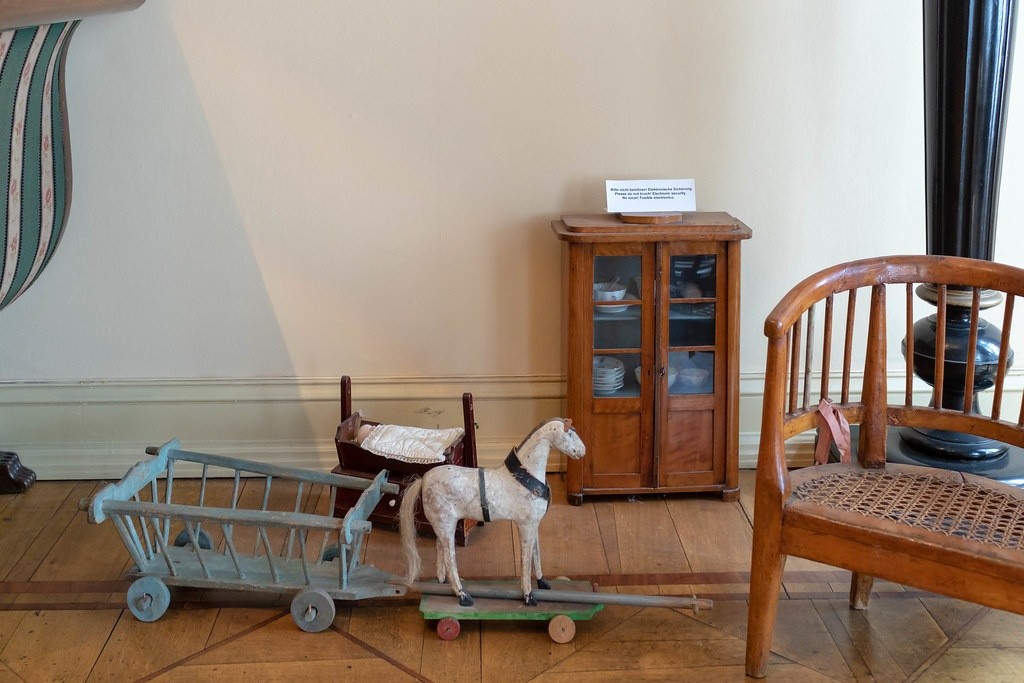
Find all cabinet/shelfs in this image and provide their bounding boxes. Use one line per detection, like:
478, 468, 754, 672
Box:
552, 210, 753, 505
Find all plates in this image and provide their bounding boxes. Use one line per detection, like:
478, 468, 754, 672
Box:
594, 356, 625, 395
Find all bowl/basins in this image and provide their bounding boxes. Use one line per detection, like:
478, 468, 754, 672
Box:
635, 365, 678, 388
594, 294, 637, 313
593, 283, 627, 301
679, 369, 710, 388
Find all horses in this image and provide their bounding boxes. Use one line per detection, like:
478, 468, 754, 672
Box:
398, 416, 587, 607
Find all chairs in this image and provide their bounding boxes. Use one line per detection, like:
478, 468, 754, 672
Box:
744, 255, 1024, 677
